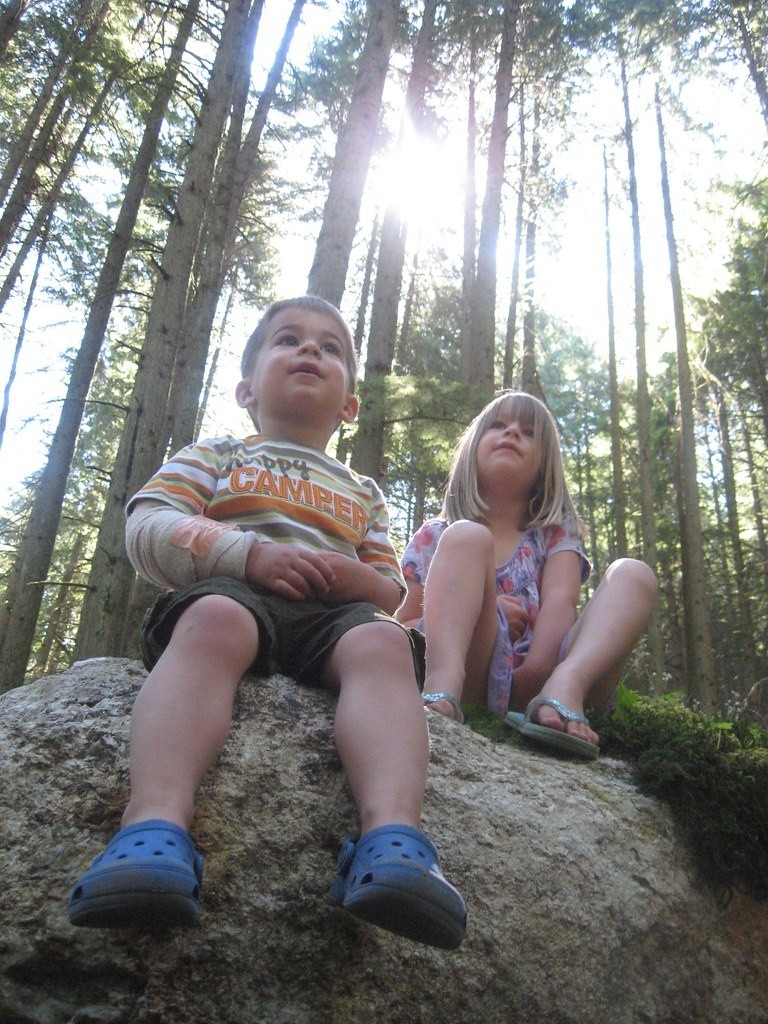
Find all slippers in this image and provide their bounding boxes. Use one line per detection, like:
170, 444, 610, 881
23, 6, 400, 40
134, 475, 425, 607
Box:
419, 693, 462, 723
504, 696, 600, 761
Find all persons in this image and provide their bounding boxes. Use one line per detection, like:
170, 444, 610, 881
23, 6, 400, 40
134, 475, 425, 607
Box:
394, 392, 659, 761
66, 294, 468, 951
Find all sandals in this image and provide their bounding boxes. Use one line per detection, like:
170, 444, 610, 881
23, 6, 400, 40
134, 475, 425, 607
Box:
328, 824, 468, 950
66, 819, 203, 930
